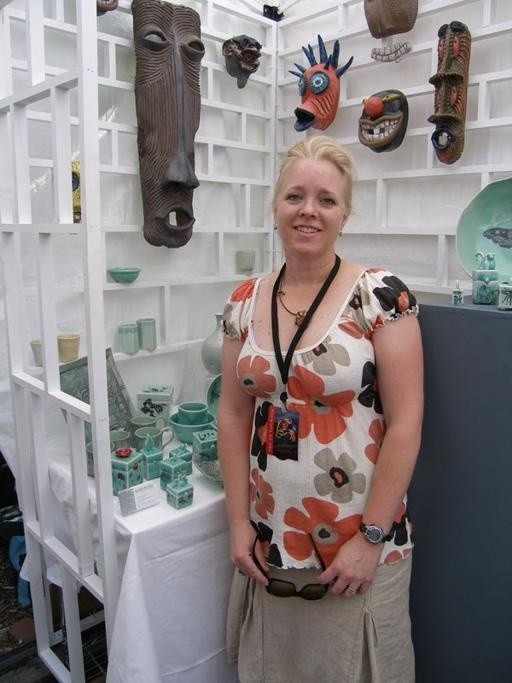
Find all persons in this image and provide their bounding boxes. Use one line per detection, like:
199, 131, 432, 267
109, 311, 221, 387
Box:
213, 132, 428, 680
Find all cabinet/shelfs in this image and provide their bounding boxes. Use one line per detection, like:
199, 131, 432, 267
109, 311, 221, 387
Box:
0, 0, 512, 683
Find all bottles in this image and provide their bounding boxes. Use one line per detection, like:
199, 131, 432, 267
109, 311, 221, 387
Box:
451, 278, 463, 306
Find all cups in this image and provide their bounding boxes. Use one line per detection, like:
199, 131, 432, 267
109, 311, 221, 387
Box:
117, 324, 139, 355
236, 249, 256, 275
30, 340, 48, 367
169, 412, 220, 444
56, 333, 80, 364
87, 415, 175, 476
177, 400, 210, 422
136, 319, 157, 352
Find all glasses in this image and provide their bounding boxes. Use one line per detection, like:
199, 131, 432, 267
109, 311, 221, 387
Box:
252, 533, 329, 600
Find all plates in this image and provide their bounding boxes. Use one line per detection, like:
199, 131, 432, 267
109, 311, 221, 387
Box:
205, 373, 223, 416
455, 177, 511, 290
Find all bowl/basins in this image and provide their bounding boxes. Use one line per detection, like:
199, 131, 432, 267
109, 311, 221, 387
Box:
108, 266, 142, 282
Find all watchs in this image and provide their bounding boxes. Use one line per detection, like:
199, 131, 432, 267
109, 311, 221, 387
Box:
357, 521, 395, 544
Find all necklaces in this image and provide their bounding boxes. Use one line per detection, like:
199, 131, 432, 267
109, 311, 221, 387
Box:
279, 268, 310, 329
